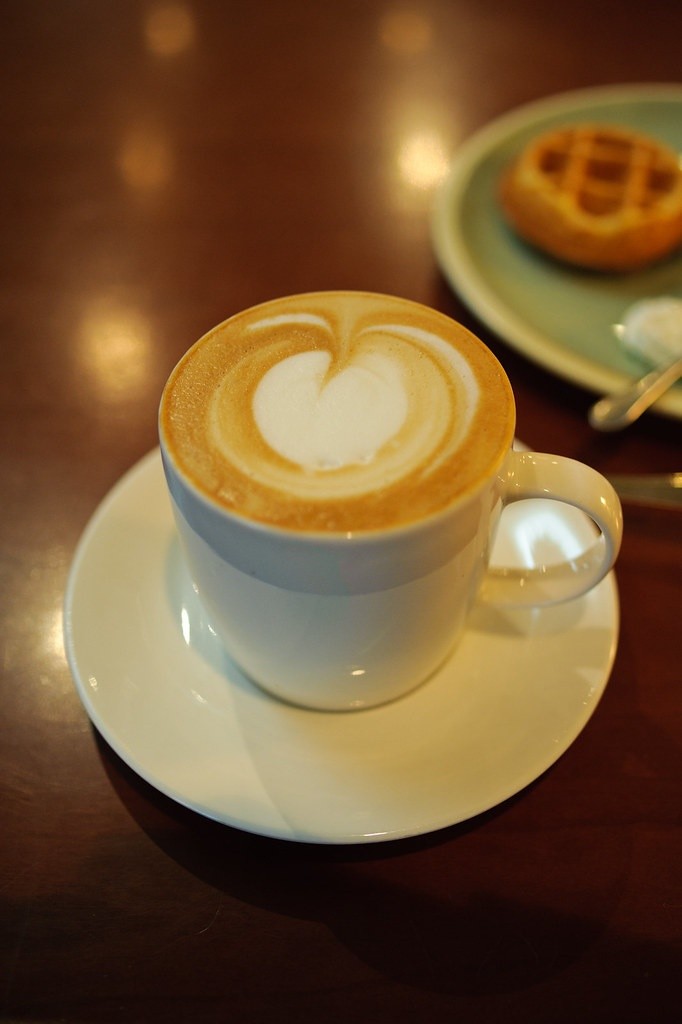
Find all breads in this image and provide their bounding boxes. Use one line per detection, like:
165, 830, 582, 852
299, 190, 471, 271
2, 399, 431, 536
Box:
501, 121, 682, 270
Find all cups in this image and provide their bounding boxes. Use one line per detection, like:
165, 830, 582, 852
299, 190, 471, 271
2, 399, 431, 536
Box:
158, 290, 623, 711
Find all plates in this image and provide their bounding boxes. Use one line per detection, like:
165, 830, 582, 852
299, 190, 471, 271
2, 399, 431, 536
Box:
430, 82, 682, 418
63, 444, 619, 844
